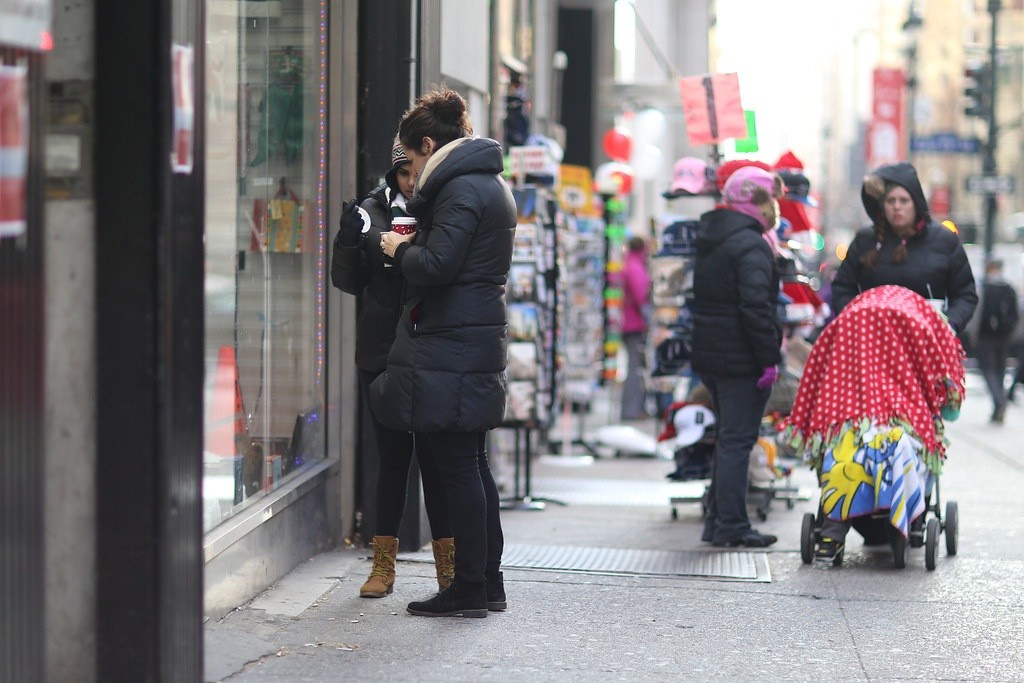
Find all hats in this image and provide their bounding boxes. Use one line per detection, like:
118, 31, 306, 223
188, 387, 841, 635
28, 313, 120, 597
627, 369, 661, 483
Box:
645, 151, 830, 491
392, 132, 409, 166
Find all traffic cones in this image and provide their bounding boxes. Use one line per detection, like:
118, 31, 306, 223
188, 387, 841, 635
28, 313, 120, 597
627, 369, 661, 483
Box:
206, 346, 248, 458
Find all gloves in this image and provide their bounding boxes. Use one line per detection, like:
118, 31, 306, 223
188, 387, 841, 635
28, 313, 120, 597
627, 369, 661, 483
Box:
756, 362, 776, 389
338, 197, 365, 247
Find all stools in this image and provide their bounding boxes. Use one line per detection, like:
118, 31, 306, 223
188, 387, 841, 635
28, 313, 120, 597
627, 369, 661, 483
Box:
496, 418, 568, 510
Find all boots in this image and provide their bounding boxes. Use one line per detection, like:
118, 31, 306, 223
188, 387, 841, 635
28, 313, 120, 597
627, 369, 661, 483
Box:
360, 535, 399, 598
432, 537, 455, 592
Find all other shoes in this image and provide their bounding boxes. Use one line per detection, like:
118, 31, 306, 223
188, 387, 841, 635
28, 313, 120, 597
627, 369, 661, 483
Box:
701, 525, 777, 547
993, 404, 1007, 423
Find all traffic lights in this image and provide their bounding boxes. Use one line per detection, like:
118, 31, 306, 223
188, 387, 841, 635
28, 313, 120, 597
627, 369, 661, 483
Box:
965, 69, 991, 114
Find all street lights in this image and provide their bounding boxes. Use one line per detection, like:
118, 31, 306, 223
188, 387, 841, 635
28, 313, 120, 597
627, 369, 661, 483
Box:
901, 0, 924, 165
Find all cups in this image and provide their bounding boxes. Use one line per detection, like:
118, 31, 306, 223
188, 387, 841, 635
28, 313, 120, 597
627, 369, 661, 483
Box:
380, 231, 397, 268
392, 216, 416, 235
926, 298, 944, 313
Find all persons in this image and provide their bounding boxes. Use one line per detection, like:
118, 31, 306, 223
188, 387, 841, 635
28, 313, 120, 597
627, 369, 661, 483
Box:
379, 86, 520, 619
973, 257, 1024, 424
687, 160, 789, 549
832, 160, 979, 547
814, 519, 851, 561
620, 235, 659, 421
330, 132, 458, 598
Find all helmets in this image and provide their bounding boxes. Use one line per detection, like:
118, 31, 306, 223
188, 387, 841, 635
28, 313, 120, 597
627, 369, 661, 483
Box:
722, 166, 785, 231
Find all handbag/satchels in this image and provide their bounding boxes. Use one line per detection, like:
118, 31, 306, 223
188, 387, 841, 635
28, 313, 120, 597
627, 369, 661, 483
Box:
251, 188, 314, 253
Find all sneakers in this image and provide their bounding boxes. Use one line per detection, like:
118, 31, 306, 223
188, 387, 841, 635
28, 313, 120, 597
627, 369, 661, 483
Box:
815, 539, 844, 562
485, 572, 507, 611
407, 574, 487, 618
